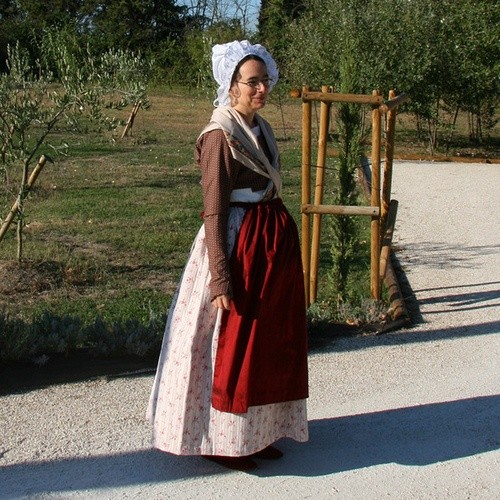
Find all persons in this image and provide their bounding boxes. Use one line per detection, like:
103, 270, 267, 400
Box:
146, 40, 309, 473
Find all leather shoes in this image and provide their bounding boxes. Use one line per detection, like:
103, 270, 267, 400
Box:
203, 456, 257, 470
255, 445, 284, 461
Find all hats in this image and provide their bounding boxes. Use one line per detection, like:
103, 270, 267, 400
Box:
211, 40, 279, 107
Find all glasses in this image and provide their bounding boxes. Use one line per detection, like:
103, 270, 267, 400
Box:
232, 78, 274, 88
